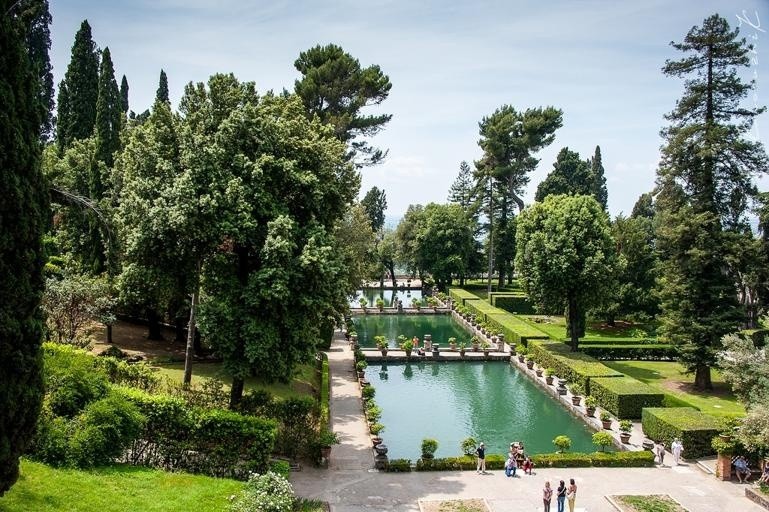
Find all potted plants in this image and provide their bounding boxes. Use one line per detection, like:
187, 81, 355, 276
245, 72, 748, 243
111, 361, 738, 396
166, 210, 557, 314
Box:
719, 432, 735, 442
511, 342, 557, 387
451, 298, 506, 345
549, 430, 618, 459
317, 428, 341, 457
418, 434, 480, 462
569, 382, 632, 444
372, 333, 490, 358
343, 302, 388, 457
358, 290, 456, 314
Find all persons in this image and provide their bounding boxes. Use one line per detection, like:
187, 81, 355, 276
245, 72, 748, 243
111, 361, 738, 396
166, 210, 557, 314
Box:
671, 438, 684, 465
734, 456, 751, 484
474, 442, 487, 474
564, 479, 577, 512
656, 440, 666, 467
505, 441, 533, 477
556, 479, 567, 512
542, 481, 553, 512
413, 335, 419, 353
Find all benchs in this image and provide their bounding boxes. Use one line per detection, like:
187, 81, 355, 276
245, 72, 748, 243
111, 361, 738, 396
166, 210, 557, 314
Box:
732, 468, 761, 474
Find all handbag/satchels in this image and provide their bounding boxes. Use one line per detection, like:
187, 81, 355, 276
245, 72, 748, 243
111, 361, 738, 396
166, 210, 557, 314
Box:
566, 491, 574, 500
